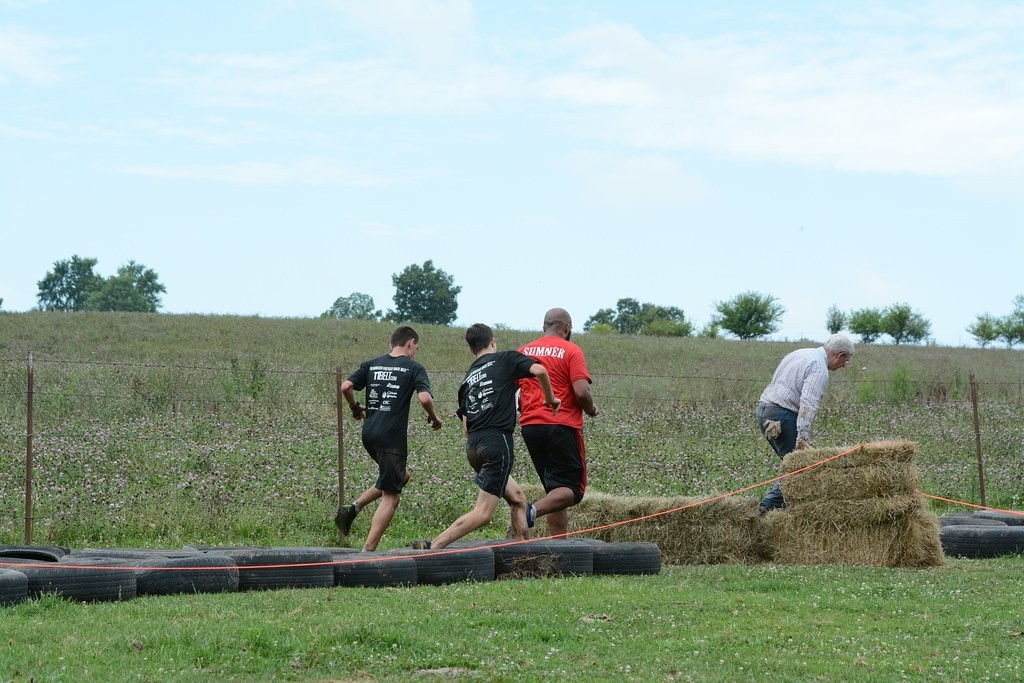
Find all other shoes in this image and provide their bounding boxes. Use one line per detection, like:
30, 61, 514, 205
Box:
759, 502, 786, 512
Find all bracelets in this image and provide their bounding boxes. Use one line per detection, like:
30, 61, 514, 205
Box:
349, 402, 359, 410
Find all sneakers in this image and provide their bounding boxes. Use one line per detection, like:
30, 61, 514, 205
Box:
412, 539, 431, 549
506, 504, 534, 540
336, 504, 357, 536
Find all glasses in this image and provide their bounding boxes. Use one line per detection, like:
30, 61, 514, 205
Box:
414, 343, 419, 350
841, 352, 850, 365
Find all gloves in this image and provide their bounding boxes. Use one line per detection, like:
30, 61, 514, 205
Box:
763, 420, 781, 440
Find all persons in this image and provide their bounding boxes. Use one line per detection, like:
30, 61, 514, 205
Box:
754, 335, 854, 515
513, 307, 599, 540
335, 326, 441, 554
414, 323, 561, 549
